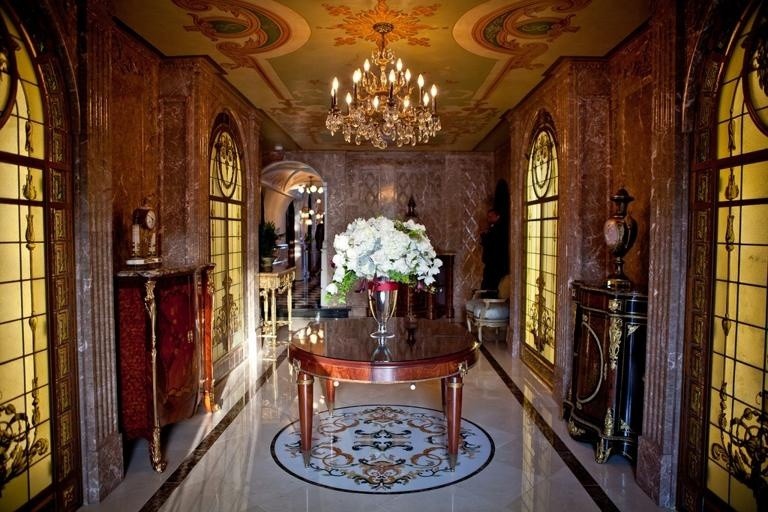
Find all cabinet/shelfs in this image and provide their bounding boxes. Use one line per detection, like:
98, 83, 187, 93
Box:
561, 279, 647, 469
112, 263, 218, 474
364, 251, 456, 320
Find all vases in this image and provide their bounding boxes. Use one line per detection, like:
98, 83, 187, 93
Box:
362, 278, 400, 343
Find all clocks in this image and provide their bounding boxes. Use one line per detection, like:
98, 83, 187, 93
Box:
124, 195, 163, 270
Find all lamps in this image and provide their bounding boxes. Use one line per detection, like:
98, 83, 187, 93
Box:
322, 22, 444, 154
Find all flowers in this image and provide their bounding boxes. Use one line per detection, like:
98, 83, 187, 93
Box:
324, 211, 447, 299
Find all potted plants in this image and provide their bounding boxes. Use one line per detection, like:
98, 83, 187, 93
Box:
258, 222, 282, 272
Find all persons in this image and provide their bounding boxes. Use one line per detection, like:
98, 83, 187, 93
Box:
473, 207, 509, 300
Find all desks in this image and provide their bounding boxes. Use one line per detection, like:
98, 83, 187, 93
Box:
282, 315, 482, 470
257, 262, 299, 346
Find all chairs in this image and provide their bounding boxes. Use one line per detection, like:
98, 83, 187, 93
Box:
462, 275, 512, 348
